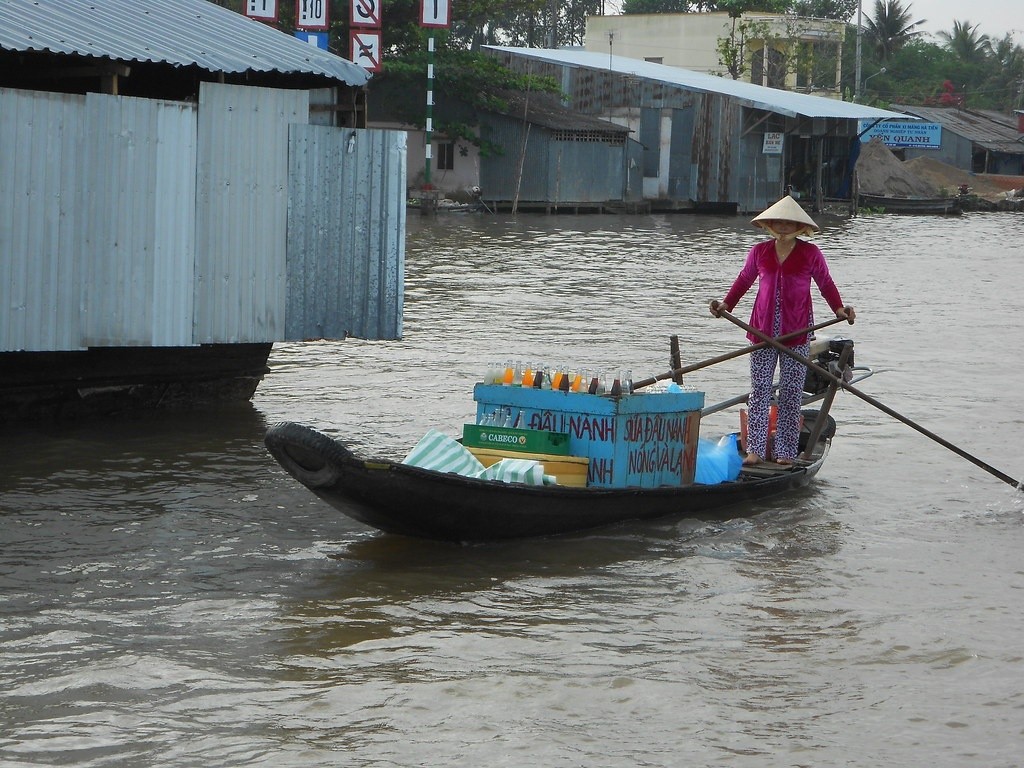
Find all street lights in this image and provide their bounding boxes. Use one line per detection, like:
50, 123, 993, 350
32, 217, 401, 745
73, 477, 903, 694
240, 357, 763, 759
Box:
864, 67, 886, 95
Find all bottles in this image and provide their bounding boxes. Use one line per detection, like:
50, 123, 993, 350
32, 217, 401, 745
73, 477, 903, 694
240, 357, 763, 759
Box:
493, 409, 501, 427
502, 415, 513, 429
500, 409, 506, 427
478, 412, 488, 425
516, 411, 526, 429
485, 414, 495, 426
485, 360, 635, 399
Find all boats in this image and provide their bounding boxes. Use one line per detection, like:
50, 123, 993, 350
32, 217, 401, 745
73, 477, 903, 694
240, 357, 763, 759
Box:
860, 191, 976, 212
977, 197, 1024, 211
407, 199, 486, 214
266, 335, 875, 535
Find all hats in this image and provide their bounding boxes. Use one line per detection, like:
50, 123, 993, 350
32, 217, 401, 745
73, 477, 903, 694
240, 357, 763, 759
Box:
750, 195, 820, 241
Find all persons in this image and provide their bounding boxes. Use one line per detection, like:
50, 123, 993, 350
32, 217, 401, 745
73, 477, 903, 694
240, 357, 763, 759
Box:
710, 195, 856, 466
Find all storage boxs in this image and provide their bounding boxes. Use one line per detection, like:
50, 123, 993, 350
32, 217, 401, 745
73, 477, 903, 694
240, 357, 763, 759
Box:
740, 406, 804, 459
474, 383, 706, 488
463, 447, 590, 488
463, 424, 571, 456
